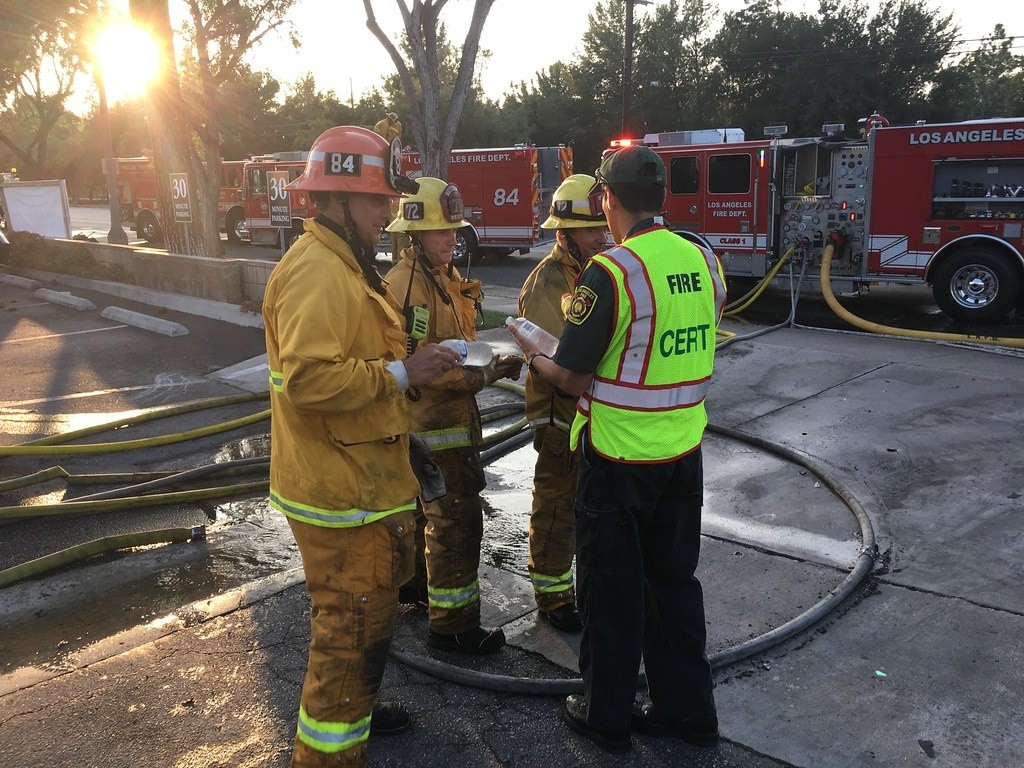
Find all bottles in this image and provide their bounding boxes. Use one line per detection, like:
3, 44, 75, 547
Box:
504, 316, 560, 359
560, 293, 574, 319
438, 338, 492, 367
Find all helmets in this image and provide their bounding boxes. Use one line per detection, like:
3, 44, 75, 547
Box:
384, 178, 470, 231
280, 125, 411, 199
539, 173, 609, 229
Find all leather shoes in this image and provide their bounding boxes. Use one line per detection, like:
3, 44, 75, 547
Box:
537, 603, 583, 635
370, 702, 412, 734
563, 692, 720, 752
427, 625, 505, 655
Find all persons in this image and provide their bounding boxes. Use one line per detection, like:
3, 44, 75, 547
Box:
262, 123, 458, 768
373, 178, 527, 658
505, 146, 728, 752
515, 172, 629, 634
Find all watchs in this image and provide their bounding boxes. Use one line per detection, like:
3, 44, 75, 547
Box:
526, 351, 550, 375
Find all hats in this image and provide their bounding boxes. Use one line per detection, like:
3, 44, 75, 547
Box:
587, 145, 667, 197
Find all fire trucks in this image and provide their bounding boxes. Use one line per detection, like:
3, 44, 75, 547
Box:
595, 109, 1021, 324
103, 156, 255, 243
243, 137, 574, 268
